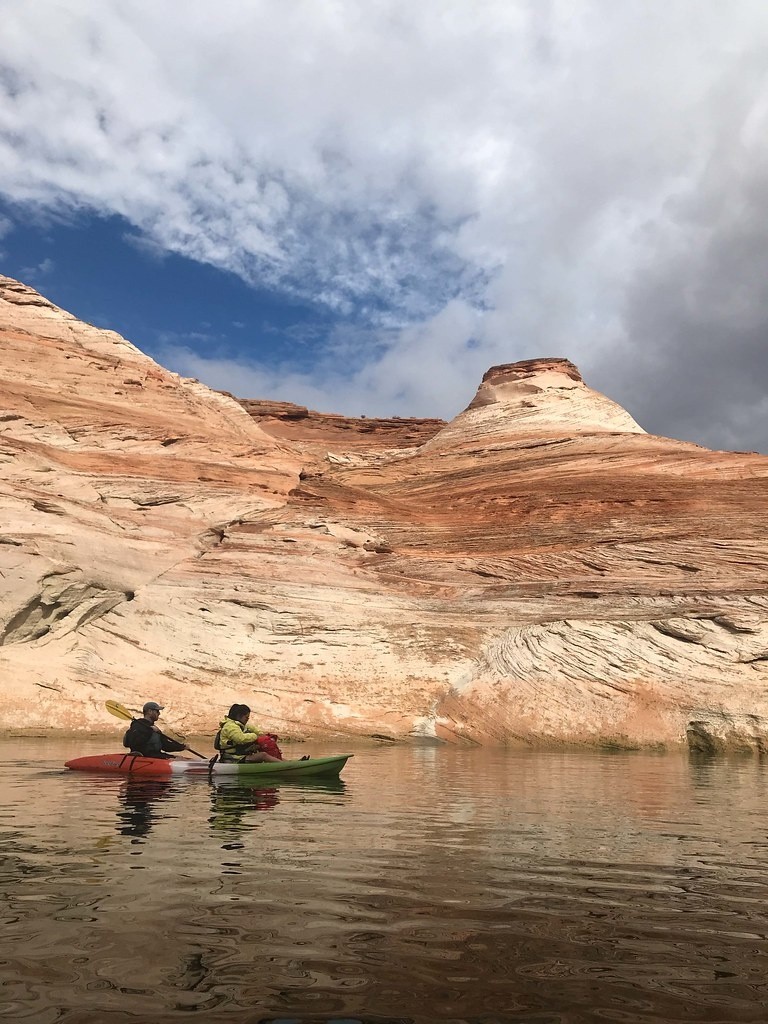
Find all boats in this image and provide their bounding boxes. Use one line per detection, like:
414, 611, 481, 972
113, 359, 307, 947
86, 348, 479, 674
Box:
63, 751, 355, 777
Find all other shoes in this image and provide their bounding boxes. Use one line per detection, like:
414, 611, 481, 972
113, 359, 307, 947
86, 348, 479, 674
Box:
298, 755, 310, 761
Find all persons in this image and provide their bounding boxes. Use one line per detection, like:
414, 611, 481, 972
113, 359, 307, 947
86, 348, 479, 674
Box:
218, 704, 311, 761
130, 703, 190, 760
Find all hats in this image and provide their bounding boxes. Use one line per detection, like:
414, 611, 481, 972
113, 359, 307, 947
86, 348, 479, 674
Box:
143, 702, 165, 711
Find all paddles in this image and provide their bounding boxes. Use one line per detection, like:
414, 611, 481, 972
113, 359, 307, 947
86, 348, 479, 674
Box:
104, 700, 208, 759
